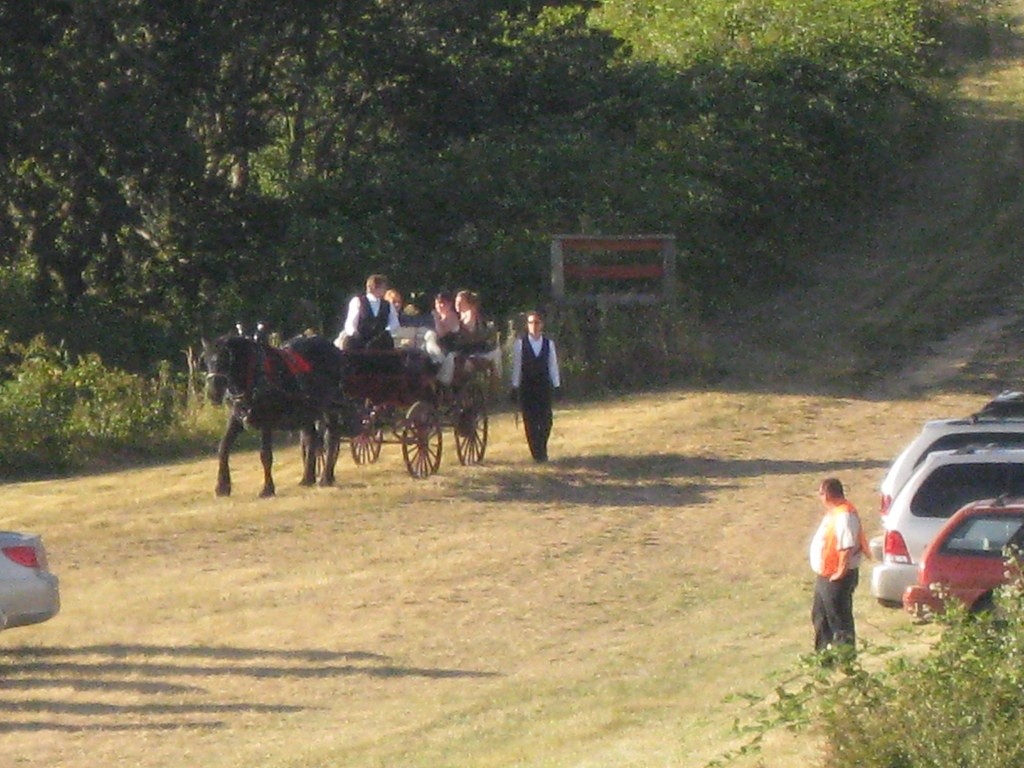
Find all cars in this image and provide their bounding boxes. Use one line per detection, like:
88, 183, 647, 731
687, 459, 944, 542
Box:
975, 390, 1024, 416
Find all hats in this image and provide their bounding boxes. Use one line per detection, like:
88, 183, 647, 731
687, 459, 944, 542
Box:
410, 291, 434, 316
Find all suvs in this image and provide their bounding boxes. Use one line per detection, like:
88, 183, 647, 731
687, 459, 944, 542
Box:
876, 416, 1023, 531
866, 443, 1024, 611
900, 493, 1024, 633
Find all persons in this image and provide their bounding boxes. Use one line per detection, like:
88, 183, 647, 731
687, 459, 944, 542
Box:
339, 274, 480, 382
808, 479, 872, 663
514, 314, 562, 463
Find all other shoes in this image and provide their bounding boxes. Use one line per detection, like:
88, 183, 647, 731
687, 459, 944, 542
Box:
817, 658, 833, 667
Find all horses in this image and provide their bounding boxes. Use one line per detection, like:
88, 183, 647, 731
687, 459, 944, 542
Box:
199, 336, 347, 498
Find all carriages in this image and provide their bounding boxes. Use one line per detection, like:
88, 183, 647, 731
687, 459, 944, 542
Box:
194, 325, 489, 501
